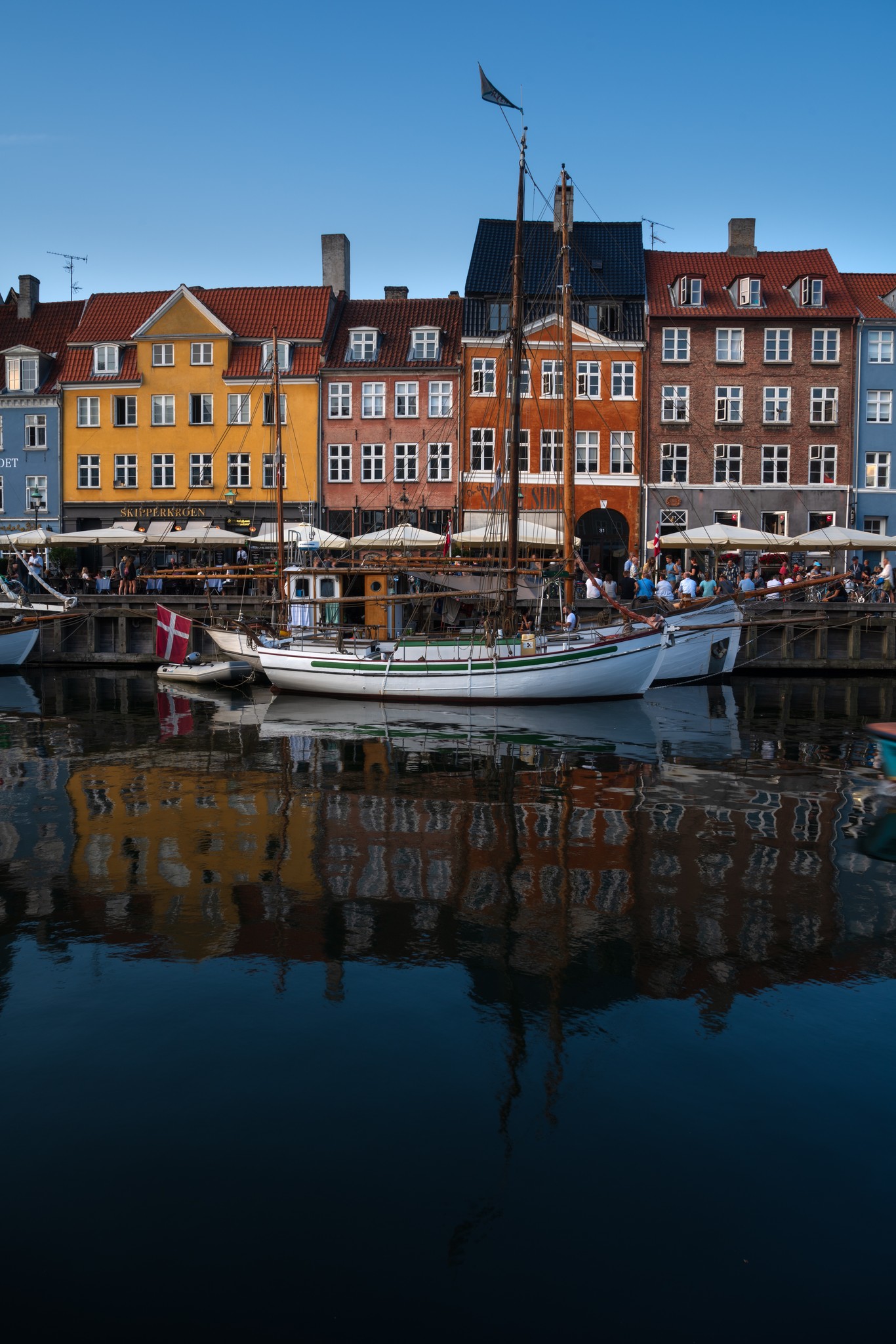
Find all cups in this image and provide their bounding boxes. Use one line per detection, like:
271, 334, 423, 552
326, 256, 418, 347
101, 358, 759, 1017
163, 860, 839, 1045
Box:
107, 577, 109, 579
640, 567, 642, 570
477, 624, 483, 629
102, 577, 105, 578
62, 576, 64, 579
68, 576, 71, 578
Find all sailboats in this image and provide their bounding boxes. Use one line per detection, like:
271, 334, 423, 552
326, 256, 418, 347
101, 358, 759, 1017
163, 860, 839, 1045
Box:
205, 123, 745, 699
0, 519, 80, 667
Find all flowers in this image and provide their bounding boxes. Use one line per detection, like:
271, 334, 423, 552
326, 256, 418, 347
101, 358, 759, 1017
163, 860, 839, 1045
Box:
717, 553, 741, 566
758, 553, 790, 565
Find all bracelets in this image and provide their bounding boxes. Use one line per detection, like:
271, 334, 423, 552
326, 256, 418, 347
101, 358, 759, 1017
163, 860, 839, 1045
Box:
111, 574, 112, 575
269, 569, 271, 572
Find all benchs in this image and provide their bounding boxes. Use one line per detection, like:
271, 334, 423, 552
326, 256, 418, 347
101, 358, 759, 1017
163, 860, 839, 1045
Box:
223, 573, 238, 596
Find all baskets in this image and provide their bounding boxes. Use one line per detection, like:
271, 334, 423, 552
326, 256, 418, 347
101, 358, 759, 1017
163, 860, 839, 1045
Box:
875, 576, 885, 586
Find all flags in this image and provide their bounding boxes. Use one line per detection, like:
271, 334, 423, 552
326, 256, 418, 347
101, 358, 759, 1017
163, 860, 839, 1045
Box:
653, 525, 661, 557
156, 603, 192, 664
443, 525, 450, 557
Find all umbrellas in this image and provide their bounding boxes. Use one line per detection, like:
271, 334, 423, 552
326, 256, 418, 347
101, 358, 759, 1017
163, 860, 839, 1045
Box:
246, 522, 358, 567
350, 523, 455, 556
146, 525, 254, 567
451, 518, 581, 567
50, 526, 156, 570
793, 524, 896, 574
0, 528, 89, 578
646, 522, 799, 583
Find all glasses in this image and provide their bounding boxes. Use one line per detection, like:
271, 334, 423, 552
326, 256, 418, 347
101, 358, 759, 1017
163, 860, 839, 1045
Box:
744, 576, 746, 577
756, 570, 759, 574
852, 559, 856, 561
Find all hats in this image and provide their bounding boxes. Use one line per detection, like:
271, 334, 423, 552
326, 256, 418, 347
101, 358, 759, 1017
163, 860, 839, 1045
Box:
814, 562, 821, 566
792, 563, 798, 568
851, 556, 859, 560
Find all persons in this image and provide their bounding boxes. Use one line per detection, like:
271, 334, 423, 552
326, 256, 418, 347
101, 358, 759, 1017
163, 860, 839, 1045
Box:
555, 606, 576, 632
79, 555, 234, 595
518, 608, 534, 636
12, 548, 67, 594
236, 547, 248, 574
619, 552, 896, 617
585, 572, 617, 599
408, 561, 446, 593
266, 556, 279, 589
313, 553, 339, 568
449, 555, 467, 576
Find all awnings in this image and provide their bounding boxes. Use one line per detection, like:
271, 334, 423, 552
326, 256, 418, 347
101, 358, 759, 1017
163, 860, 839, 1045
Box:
185, 521, 212, 530
112, 522, 138, 530
462, 511, 575, 532
146, 521, 175, 535
257, 523, 301, 537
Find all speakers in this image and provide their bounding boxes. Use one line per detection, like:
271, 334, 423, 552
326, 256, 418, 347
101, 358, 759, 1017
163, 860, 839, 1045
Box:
364, 575, 403, 641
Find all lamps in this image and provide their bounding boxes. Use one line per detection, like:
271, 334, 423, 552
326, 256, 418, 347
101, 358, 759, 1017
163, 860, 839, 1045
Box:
223, 489, 241, 517
102, 546, 109, 548
322, 508, 325, 513
355, 509, 358, 513
123, 502, 131, 512
215, 526, 220, 529
187, 502, 194, 511
454, 508, 456, 513
249, 527, 256, 532
155, 502, 163, 512
46, 523, 53, 532
139, 502, 146, 512
138, 527, 145, 532
175, 526, 182, 531
388, 508, 391, 513
172, 502, 178, 512
421, 508, 424, 512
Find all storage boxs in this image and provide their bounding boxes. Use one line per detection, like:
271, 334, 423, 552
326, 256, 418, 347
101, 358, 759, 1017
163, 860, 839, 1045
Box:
582, 573, 595, 581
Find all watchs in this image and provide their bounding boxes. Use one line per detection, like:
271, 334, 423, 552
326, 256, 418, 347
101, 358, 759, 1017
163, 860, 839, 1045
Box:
35, 564, 36, 566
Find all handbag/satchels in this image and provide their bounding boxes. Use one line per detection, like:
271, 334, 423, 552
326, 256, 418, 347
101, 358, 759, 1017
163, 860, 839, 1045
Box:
237, 560, 244, 565
124, 567, 129, 575
698, 577, 702, 581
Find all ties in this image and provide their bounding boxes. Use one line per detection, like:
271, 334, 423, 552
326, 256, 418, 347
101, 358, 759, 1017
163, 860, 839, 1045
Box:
240, 551, 242, 558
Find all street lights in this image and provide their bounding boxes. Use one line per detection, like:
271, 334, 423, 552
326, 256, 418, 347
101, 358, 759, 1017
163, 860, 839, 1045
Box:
29, 485, 43, 531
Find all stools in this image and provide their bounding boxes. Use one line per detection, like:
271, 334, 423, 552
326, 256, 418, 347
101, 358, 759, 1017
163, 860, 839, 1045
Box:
275, 578, 279, 590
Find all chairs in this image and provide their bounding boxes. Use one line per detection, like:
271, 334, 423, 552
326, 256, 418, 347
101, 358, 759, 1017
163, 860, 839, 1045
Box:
191, 579, 205, 595
39, 578, 51, 594
81, 578, 97, 594
48, 578, 67, 594
163, 578, 183, 595
110, 578, 122, 595
67, 578, 84, 594
574, 615, 580, 631
136, 579, 147, 595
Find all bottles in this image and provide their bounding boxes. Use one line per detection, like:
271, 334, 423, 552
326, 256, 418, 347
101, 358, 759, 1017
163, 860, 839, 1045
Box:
105, 573, 107, 579
193, 563, 205, 569
397, 631, 400, 638
220, 572, 221, 575
362, 630, 365, 639
213, 572, 217, 575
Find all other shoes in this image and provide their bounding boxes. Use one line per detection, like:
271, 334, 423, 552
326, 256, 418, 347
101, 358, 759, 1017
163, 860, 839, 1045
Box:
271, 583, 277, 589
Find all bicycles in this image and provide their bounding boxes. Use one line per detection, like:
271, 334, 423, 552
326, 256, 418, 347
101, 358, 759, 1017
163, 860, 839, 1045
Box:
808, 585, 823, 602
844, 573, 891, 603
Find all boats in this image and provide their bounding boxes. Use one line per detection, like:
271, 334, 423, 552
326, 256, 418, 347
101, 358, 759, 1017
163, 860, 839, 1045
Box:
158, 654, 252, 686
863, 723, 896, 783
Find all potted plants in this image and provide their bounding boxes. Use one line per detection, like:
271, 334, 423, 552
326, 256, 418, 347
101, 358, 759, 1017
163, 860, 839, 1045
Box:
406, 628, 415, 636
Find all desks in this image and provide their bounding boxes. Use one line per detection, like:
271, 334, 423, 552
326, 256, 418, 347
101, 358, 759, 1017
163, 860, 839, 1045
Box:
204, 578, 226, 595
146, 578, 165, 595
256, 571, 272, 596
96, 578, 115, 595
